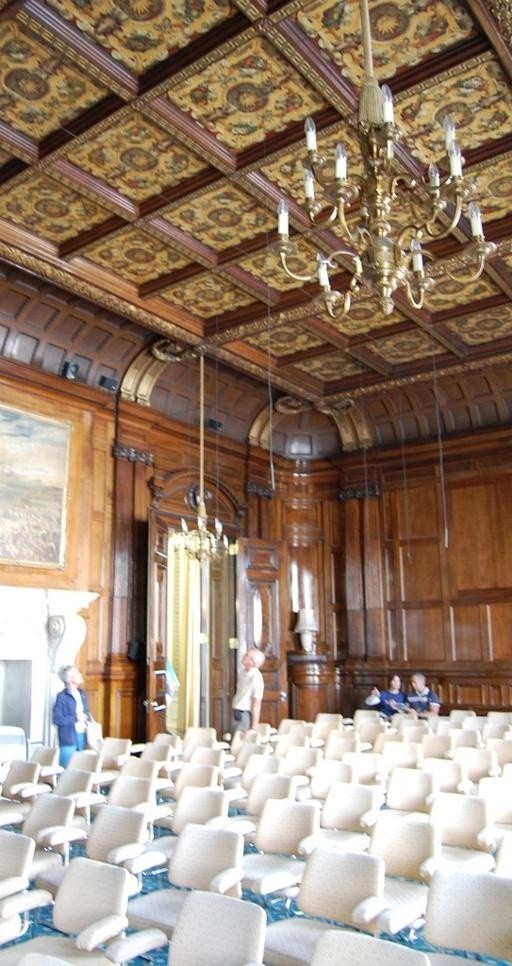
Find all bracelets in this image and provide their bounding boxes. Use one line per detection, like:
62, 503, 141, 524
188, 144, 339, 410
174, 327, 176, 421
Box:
251, 728, 257, 731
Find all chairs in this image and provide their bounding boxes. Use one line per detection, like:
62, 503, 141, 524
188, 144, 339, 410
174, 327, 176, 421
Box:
0, 710, 510, 966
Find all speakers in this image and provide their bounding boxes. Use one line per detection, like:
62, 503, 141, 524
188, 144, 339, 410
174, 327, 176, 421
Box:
99, 375, 118, 391
208, 419, 222, 429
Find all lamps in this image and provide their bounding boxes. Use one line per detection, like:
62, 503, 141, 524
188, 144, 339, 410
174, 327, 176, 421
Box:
292, 606, 323, 657
160, 344, 240, 567
274, 0, 498, 324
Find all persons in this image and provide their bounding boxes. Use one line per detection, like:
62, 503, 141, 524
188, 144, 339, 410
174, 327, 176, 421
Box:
389, 672, 440, 721
231, 649, 266, 737
52, 667, 91, 768
371, 673, 407, 718
